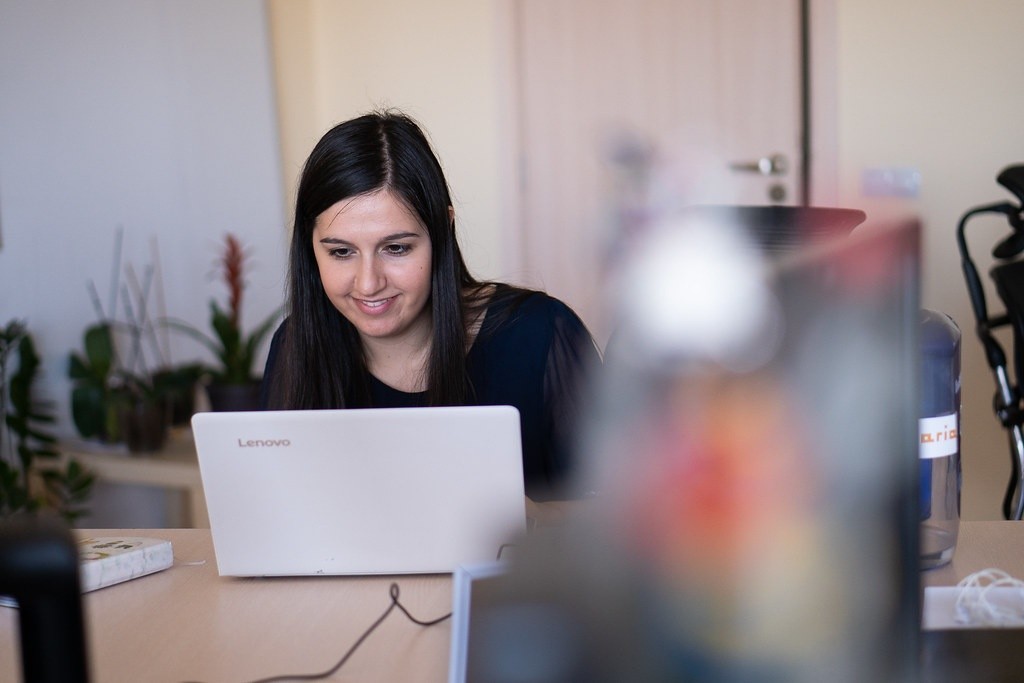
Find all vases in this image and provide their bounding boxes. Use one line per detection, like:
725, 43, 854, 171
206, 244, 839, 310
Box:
201, 378, 262, 412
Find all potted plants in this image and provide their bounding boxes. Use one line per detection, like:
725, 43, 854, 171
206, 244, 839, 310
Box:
66, 319, 208, 455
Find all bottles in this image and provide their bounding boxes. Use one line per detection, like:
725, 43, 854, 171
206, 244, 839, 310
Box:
917, 307, 963, 573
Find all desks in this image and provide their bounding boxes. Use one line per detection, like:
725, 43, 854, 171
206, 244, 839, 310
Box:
1, 519, 1024, 683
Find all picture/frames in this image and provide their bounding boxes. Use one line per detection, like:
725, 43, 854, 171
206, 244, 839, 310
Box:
451, 560, 509, 683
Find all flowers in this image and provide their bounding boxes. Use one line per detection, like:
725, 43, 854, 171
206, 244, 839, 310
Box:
156, 233, 289, 377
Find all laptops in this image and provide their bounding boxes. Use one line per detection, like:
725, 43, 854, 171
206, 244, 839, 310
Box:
191, 405, 527, 577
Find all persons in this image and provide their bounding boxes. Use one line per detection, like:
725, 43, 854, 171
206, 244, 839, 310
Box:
258, 104, 605, 526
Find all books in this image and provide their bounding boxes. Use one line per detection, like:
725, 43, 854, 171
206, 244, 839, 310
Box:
0, 533, 174, 608
